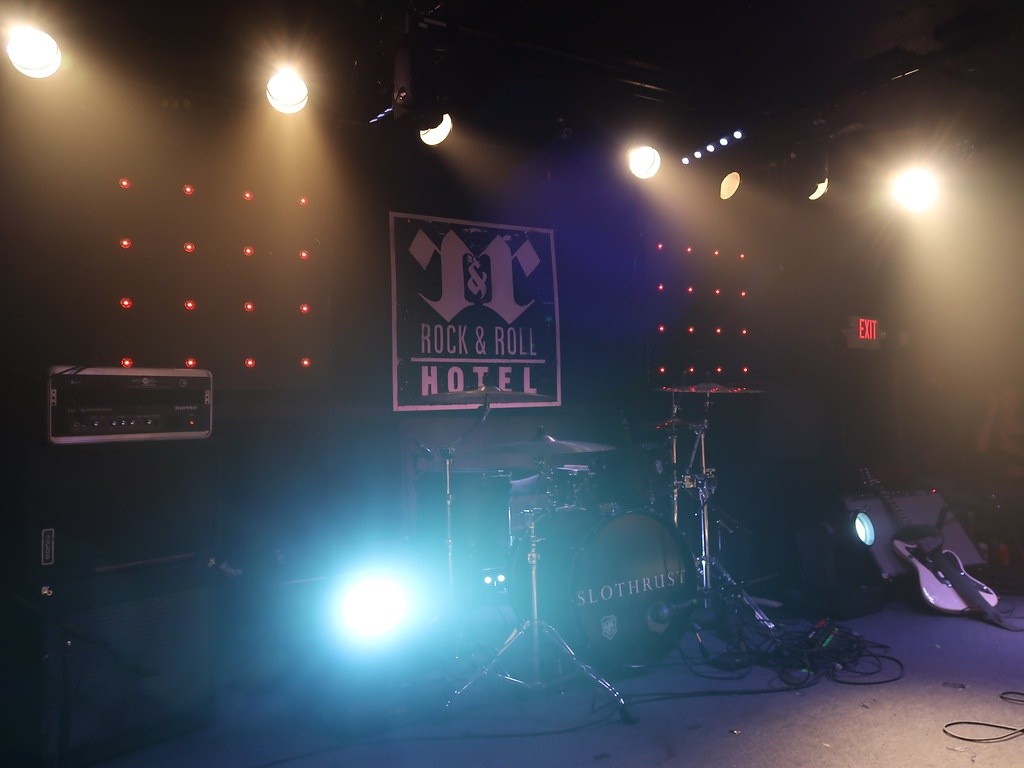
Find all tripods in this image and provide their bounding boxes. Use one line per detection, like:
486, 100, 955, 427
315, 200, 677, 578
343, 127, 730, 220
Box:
694, 395, 787, 648
445, 457, 641, 728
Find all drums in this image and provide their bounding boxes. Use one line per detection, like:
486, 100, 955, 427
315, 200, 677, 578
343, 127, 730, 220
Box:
509, 507, 697, 669
425, 470, 511, 538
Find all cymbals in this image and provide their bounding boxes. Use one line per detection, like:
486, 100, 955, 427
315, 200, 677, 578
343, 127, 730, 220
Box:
659, 383, 766, 393
479, 435, 618, 455
644, 418, 703, 426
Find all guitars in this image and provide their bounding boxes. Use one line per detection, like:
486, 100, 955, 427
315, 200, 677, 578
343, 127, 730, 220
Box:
858, 467, 999, 615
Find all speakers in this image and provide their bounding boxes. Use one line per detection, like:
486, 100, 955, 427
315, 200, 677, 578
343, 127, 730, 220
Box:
34, 547, 216, 768
